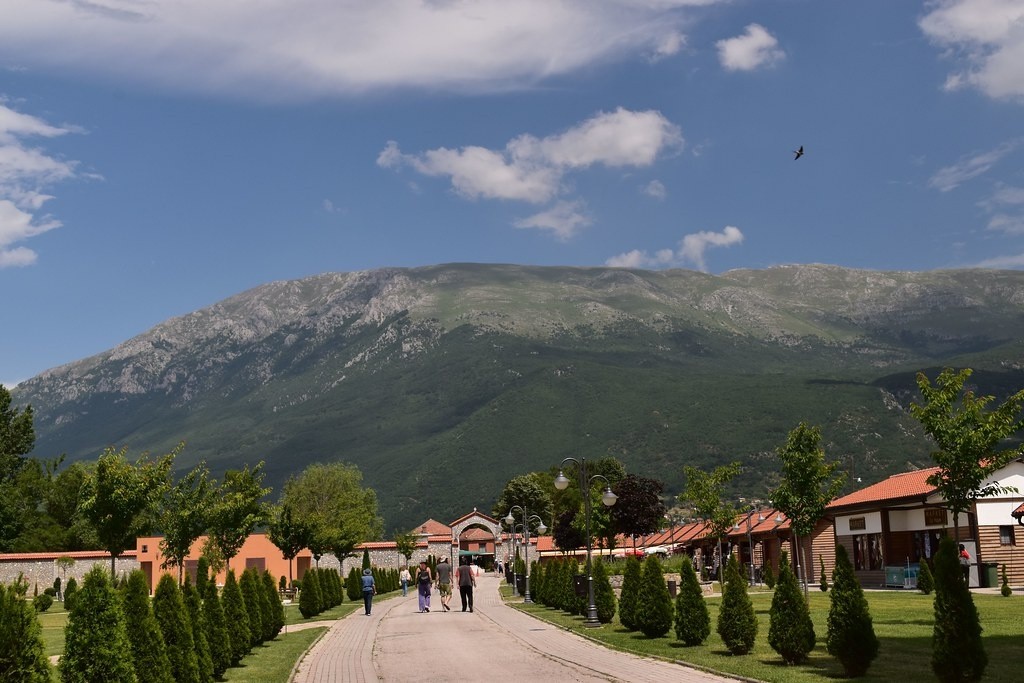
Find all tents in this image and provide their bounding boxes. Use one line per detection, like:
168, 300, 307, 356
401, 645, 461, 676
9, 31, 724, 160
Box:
459, 550, 479, 557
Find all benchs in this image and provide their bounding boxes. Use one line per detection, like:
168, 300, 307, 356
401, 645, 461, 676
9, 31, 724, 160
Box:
278, 588, 297, 601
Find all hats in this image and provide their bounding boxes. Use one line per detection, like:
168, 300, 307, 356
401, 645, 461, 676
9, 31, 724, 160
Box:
440, 555, 449, 562
363, 569, 372, 576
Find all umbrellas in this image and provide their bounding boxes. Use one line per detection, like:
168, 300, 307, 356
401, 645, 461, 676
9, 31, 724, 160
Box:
615, 549, 644, 558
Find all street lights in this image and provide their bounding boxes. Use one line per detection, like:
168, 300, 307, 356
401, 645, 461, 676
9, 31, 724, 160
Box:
773, 509, 804, 592
505, 505, 549, 604
733, 509, 766, 589
553, 457, 619, 630
496, 517, 531, 597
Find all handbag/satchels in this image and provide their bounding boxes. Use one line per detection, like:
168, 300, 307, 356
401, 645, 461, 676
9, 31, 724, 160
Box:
406, 571, 411, 580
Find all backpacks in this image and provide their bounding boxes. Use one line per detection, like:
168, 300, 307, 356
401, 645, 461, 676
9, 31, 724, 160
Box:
418, 566, 430, 584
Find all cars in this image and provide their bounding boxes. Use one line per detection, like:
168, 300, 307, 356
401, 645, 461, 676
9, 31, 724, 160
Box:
625, 542, 687, 560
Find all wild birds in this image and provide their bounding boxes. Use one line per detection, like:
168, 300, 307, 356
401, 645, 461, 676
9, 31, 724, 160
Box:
792, 144, 805, 161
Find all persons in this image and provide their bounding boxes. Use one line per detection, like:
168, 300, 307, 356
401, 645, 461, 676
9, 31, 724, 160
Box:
460, 556, 467, 565
415, 559, 433, 613
434, 555, 454, 612
957, 544, 970, 591
493, 558, 509, 578
398, 565, 412, 597
455, 557, 477, 613
362, 569, 377, 615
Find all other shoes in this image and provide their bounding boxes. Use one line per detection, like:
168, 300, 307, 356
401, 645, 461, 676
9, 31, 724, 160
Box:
425, 605, 431, 612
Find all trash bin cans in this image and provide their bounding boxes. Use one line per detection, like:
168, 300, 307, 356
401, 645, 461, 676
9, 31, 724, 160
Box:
507, 571, 514, 584
668, 577, 677, 599
516, 574, 524, 589
574, 574, 587, 599
505, 569, 509, 577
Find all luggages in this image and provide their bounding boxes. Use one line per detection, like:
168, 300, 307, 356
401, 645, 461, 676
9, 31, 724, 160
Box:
443, 603, 450, 612
